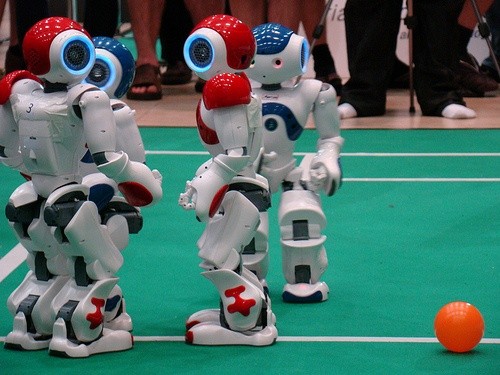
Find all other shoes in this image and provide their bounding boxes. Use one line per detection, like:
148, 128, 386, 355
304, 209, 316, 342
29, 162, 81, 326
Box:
159, 64, 193, 85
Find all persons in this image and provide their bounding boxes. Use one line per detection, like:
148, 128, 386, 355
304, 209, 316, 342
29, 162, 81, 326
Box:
179, 15, 277, 347
81, 36, 146, 332
0, 0, 492, 120
0, 16, 163, 357
249, 24, 345, 303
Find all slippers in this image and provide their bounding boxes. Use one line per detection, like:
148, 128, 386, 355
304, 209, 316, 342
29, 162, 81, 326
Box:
127, 64, 163, 100
